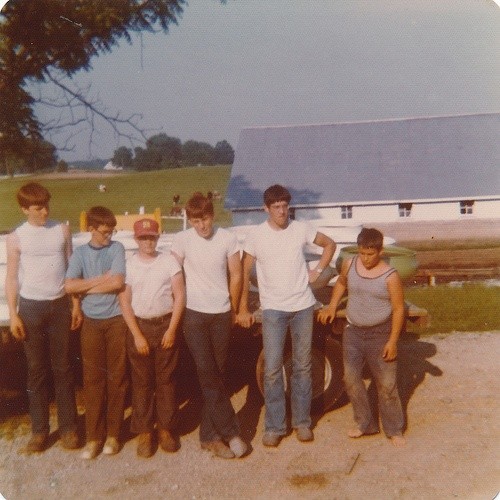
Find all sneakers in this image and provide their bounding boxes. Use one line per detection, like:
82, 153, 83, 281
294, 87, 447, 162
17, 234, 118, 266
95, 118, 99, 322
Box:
229, 438, 248, 458
158, 429, 181, 451
104, 436, 120, 454
263, 433, 285, 446
59, 433, 79, 449
137, 435, 153, 458
200, 439, 234, 460
295, 427, 315, 442
28, 433, 47, 450
80, 440, 103, 458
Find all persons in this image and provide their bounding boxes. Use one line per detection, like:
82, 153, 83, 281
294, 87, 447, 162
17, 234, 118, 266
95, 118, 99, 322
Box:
316, 228, 409, 446
237, 184, 336, 447
7, 182, 82, 452
118, 218, 187, 458
170, 196, 248, 459
64, 206, 127, 459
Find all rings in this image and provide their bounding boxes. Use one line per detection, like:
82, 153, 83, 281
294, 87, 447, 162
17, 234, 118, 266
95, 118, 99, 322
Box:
321, 316, 324, 320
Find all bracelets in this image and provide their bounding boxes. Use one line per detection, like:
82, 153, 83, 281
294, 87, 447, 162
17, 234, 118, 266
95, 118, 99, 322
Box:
315, 266, 323, 273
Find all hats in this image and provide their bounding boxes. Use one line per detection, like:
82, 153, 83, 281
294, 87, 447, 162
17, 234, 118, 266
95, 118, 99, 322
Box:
133, 220, 160, 238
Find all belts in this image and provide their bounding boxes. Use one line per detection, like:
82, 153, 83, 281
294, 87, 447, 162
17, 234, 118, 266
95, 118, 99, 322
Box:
136, 313, 172, 324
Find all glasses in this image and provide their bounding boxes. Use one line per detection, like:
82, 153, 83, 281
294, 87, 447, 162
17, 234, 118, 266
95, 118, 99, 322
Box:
92, 225, 117, 237
